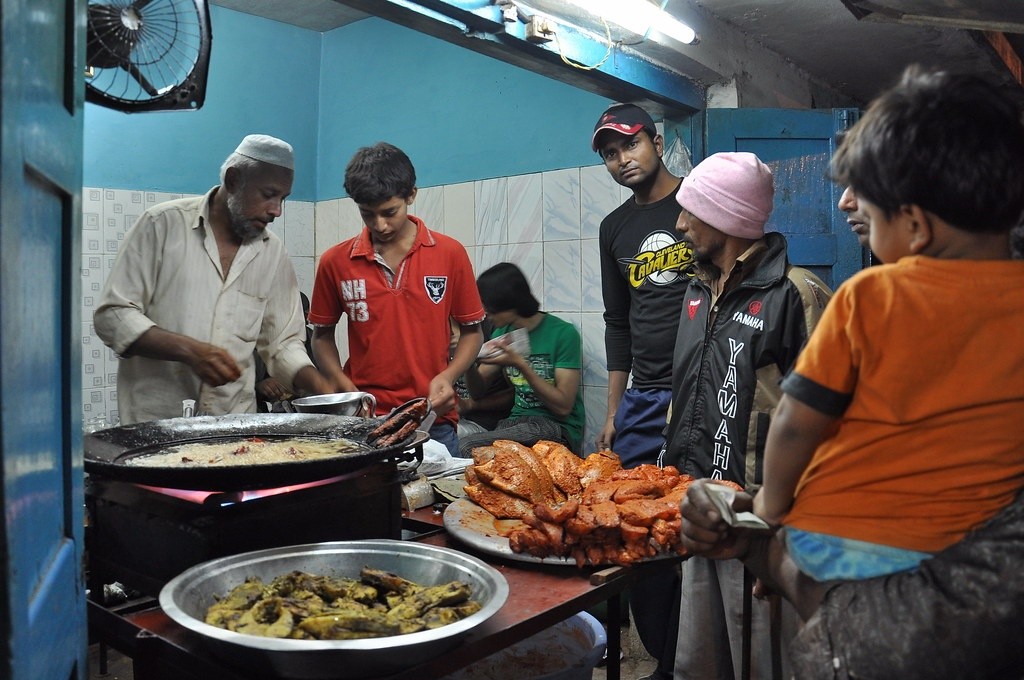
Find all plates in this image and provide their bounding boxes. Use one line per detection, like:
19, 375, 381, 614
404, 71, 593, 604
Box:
443, 498, 683, 565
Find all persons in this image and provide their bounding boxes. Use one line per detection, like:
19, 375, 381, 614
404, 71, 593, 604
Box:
94, 135, 335, 426
449, 263, 585, 459
591, 105, 696, 679
307, 142, 486, 456
679, 63, 1023, 679
253, 292, 314, 413
657, 151, 834, 679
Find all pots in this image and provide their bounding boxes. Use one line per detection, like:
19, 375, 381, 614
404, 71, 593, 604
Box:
84, 398, 432, 488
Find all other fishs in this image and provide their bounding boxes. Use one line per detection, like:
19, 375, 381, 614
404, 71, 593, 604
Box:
463, 438, 744, 569
204, 565, 483, 639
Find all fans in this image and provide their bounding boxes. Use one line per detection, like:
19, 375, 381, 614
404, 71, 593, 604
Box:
86, 0, 213, 112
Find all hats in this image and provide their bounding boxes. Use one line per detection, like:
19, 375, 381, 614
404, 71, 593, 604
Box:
235, 133, 294, 171
592, 101, 657, 152
674, 149, 774, 240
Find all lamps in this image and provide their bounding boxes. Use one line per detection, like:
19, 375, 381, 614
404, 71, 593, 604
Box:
571, 0, 702, 45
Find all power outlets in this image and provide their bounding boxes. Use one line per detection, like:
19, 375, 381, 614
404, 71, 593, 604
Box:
525, 15, 554, 42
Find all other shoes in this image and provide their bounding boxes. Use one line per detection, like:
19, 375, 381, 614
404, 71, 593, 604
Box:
636, 669, 673, 680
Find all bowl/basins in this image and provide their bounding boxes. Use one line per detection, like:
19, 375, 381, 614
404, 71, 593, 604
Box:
292, 391, 377, 419
159, 538, 510, 652
440, 610, 607, 680
